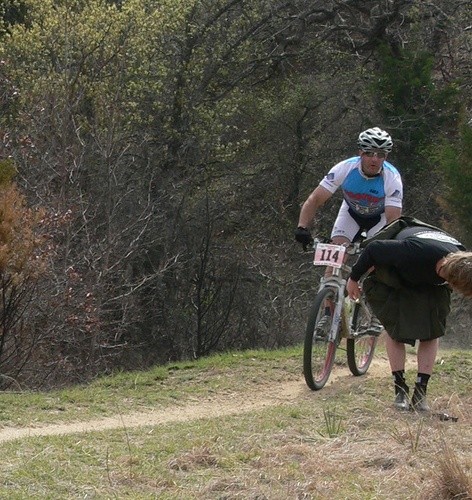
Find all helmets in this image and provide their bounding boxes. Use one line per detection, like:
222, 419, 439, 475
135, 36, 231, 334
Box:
357, 127, 394, 152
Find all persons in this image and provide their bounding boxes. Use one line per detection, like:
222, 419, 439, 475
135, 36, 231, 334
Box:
295, 127, 405, 342
347, 215, 472, 410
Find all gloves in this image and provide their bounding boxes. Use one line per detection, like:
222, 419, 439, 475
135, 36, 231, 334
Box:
294, 226, 312, 251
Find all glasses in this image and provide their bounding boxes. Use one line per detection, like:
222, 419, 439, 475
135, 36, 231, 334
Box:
360, 149, 385, 158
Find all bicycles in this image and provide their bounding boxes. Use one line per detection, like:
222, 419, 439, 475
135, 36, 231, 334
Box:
302, 237, 384, 392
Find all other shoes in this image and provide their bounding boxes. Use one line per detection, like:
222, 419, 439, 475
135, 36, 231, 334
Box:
315, 315, 332, 337
366, 313, 384, 334
410, 385, 429, 411
389, 384, 409, 410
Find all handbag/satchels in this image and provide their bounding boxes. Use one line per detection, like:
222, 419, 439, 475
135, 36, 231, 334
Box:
360, 214, 415, 249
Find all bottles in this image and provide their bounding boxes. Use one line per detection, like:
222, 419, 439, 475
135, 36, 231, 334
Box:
430, 413, 458, 422
344, 298, 352, 317
